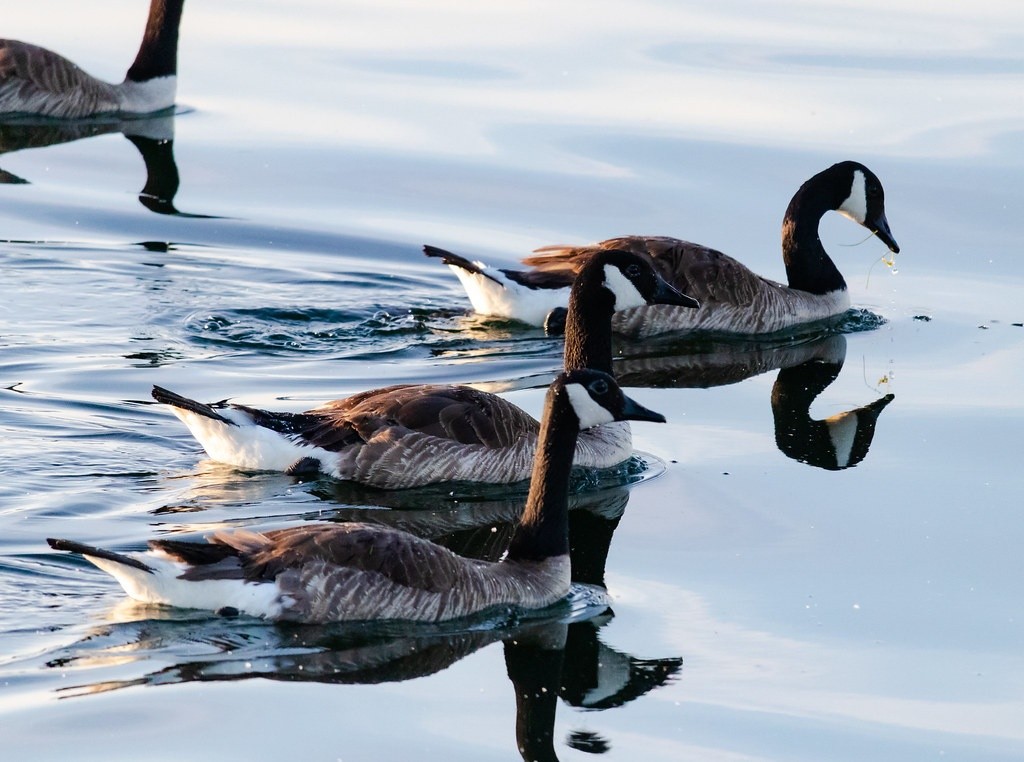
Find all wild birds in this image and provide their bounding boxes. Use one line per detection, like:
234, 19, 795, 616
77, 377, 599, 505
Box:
524, 327, 901, 473
0, 0, 185, 118
51, 613, 569, 762
149, 247, 702, 490
421, 159, 899, 338
142, 465, 682, 705
0, 113, 225, 219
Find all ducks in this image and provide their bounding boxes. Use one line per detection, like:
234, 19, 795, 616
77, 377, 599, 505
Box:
45, 367, 668, 627
